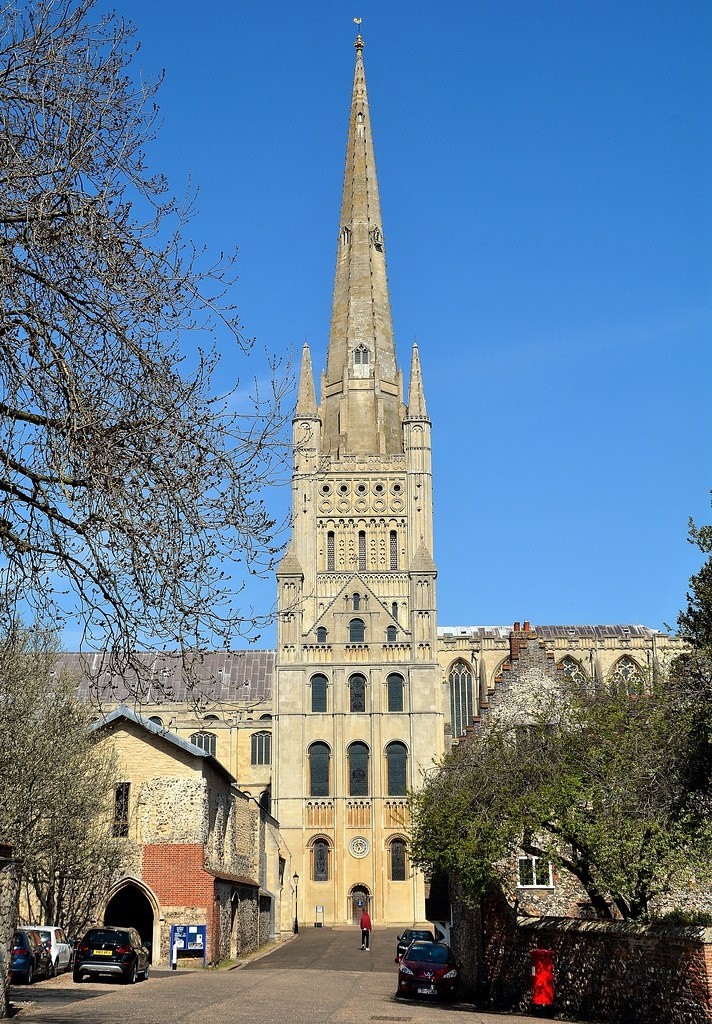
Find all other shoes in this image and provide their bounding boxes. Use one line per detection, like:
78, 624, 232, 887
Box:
366, 948, 370, 951
361, 944, 364, 950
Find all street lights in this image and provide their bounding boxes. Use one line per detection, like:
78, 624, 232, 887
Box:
292, 871, 300, 934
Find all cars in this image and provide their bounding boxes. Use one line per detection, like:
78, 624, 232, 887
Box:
396, 928, 440, 959
10, 930, 54, 985
394, 939, 465, 1003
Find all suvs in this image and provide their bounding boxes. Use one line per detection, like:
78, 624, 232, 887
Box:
16, 925, 76, 977
73, 925, 152, 984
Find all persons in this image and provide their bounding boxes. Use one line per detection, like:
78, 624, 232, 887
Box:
360, 908, 372, 951
